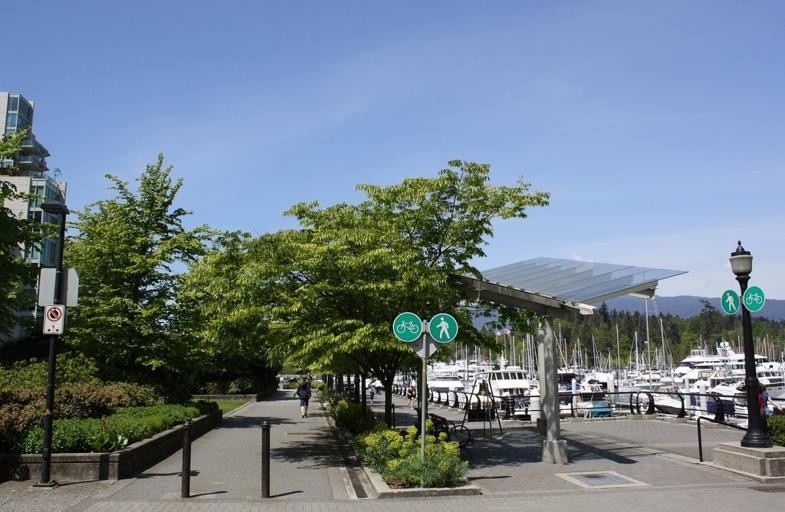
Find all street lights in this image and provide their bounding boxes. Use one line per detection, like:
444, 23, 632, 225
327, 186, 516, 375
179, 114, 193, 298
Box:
730, 237, 775, 452
30, 200, 69, 487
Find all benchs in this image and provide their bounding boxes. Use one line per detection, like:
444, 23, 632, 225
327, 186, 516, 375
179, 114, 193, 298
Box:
414, 407, 473, 460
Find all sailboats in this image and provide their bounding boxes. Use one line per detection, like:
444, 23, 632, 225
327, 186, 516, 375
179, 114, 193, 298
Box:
343, 296, 785, 421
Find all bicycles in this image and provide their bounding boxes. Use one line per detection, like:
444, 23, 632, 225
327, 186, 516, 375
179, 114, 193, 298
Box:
412, 416, 473, 448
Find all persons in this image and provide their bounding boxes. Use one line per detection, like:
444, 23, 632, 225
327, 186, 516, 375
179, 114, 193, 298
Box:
763, 384, 782, 415
759, 383, 765, 416
407, 383, 415, 411
369, 384, 375, 404
297, 376, 311, 417
